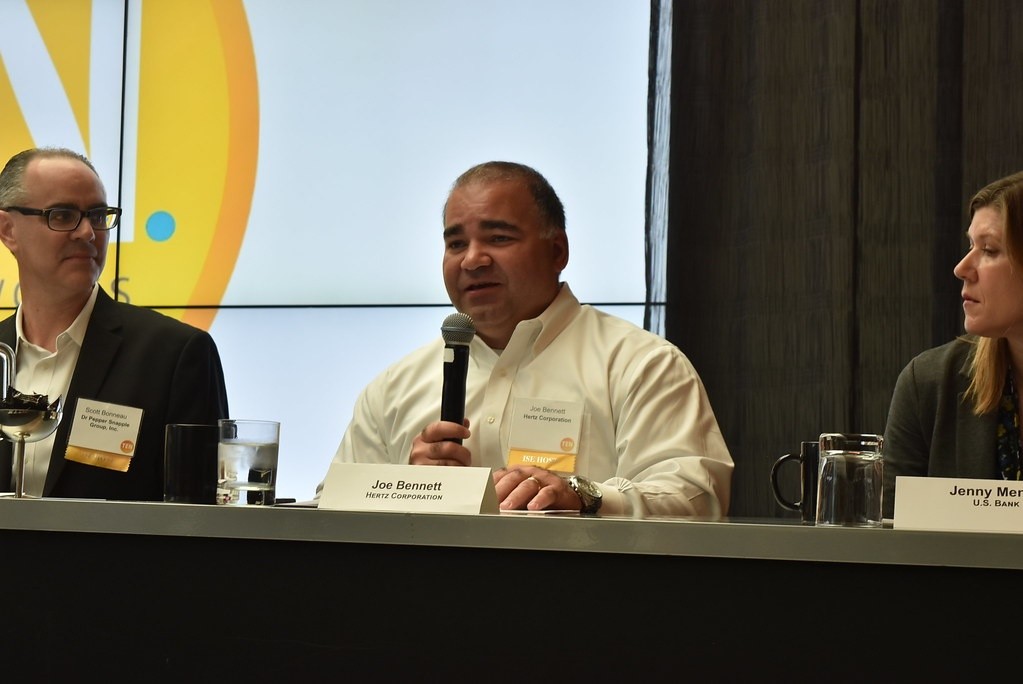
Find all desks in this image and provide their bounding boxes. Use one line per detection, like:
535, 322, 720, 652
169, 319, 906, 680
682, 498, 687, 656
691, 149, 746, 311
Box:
1, 496, 1023, 684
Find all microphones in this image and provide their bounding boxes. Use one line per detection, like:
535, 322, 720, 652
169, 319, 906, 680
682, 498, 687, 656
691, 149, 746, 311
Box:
440, 313, 478, 446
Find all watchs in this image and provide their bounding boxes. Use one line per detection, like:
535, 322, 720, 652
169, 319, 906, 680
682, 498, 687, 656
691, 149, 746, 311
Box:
561, 475, 603, 515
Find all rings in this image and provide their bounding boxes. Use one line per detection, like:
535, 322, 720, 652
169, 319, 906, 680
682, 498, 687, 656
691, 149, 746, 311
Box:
528, 477, 542, 490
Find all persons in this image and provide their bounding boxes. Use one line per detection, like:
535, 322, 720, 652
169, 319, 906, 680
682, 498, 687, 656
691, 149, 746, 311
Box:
882, 172, 1023, 519
0, 148, 230, 505
313, 160, 734, 519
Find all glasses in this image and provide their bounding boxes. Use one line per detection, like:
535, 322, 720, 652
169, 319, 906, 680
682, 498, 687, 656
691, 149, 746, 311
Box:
0, 206, 122, 232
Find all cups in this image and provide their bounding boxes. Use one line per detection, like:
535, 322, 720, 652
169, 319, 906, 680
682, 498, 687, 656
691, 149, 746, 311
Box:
161, 423, 221, 503
1, 395, 65, 498
217, 418, 279, 508
772, 433, 887, 529
